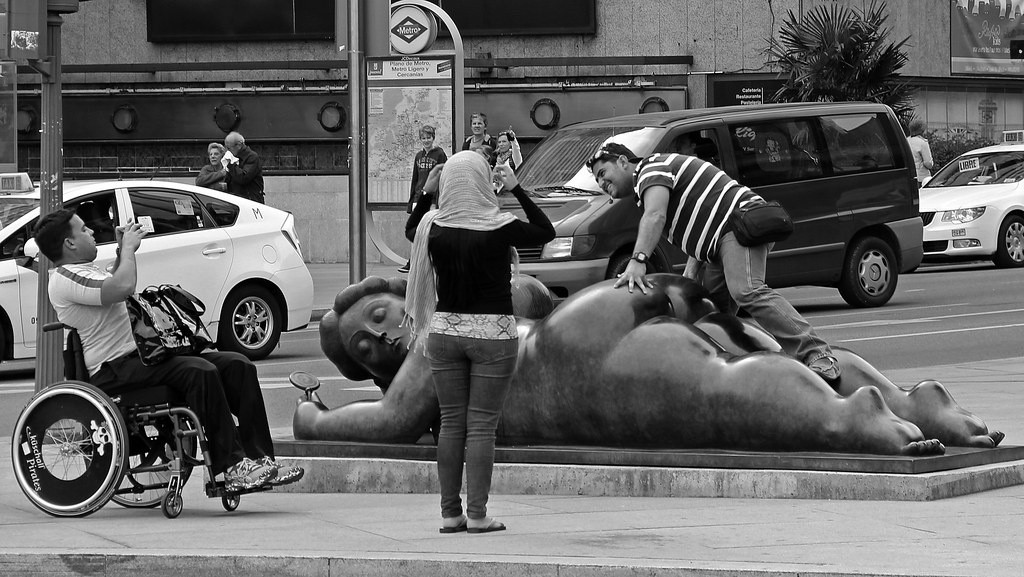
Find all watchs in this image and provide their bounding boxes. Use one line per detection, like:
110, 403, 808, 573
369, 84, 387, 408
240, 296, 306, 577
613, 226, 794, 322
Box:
631, 253, 650, 265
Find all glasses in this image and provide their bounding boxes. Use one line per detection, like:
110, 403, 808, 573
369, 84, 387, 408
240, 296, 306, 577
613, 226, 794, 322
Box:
586, 150, 620, 165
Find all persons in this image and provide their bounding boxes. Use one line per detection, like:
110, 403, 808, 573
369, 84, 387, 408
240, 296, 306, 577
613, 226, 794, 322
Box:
586, 142, 841, 380
195, 131, 264, 204
858, 155, 879, 171
33, 207, 304, 492
292, 272, 1005, 455
675, 135, 714, 165
398, 125, 446, 274
906, 119, 934, 189
406, 150, 556, 533
461, 112, 523, 197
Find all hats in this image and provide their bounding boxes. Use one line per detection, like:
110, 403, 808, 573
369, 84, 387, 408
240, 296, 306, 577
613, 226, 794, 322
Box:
909, 119, 927, 137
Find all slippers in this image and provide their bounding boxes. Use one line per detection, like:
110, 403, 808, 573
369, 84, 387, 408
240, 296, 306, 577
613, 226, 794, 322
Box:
439, 515, 506, 533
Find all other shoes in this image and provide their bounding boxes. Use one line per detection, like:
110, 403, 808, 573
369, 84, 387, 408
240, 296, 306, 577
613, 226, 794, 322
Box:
807, 357, 842, 379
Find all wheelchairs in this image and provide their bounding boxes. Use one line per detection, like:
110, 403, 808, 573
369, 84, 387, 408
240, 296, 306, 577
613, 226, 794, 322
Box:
10, 320, 274, 520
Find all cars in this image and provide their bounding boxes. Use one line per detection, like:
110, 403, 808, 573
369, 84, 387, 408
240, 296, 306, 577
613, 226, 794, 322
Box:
919, 143, 1024, 268
0, 181, 314, 363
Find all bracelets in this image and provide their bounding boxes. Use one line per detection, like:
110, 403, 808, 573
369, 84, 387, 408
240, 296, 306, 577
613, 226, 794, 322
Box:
116, 246, 120, 259
420, 188, 436, 198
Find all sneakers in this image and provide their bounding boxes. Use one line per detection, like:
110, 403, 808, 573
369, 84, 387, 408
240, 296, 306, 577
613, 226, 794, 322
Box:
224, 455, 304, 493
397, 259, 410, 272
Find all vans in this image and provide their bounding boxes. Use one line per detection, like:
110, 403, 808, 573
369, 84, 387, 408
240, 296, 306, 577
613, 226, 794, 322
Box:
498, 101, 923, 310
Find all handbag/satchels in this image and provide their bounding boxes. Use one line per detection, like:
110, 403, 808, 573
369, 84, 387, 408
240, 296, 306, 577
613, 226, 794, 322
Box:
124, 283, 213, 366
727, 201, 793, 247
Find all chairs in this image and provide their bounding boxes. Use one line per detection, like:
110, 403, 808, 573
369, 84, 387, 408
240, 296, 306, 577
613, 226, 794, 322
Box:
76, 202, 113, 241
751, 125, 795, 183
